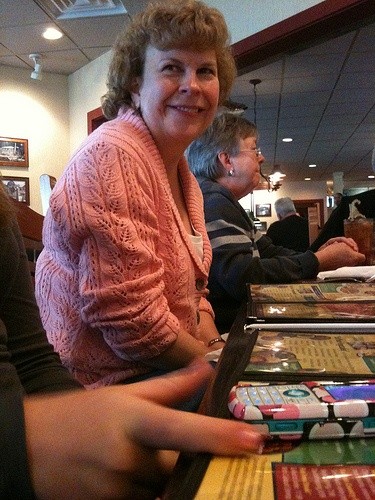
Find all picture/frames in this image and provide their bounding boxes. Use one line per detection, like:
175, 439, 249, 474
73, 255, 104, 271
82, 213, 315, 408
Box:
0, 176, 30, 206
0, 137, 29, 167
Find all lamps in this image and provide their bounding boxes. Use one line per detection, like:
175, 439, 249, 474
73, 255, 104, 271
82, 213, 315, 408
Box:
29, 53, 42, 81
267, 163, 286, 183
248, 79, 281, 190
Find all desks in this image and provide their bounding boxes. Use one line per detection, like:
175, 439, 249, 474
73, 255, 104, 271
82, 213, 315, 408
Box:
179, 275, 375, 500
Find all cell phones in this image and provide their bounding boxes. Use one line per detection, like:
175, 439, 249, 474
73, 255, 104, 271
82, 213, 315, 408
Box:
225, 377, 375, 442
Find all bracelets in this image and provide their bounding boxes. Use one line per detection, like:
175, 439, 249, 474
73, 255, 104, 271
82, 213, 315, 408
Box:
207, 338, 226, 347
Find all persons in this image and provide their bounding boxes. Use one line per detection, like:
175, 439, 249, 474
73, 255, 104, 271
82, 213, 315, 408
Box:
184, 114, 367, 333
265, 197, 310, 252
0, 176, 260, 500
33, 0, 236, 390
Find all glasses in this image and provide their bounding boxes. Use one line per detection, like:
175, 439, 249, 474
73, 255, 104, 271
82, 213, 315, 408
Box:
240, 147, 261, 157
